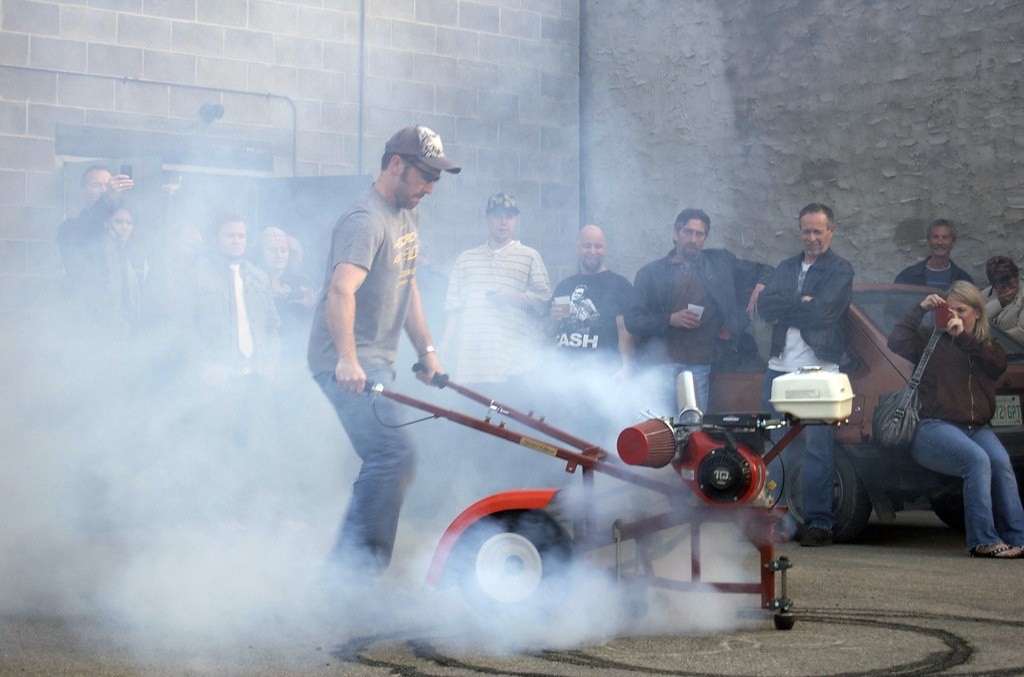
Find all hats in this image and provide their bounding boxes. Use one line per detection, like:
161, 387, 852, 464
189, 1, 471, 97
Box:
384, 124, 463, 173
486, 193, 520, 215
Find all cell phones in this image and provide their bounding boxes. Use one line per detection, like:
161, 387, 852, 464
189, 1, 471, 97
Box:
934, 302, 950, 326
120, 165, 133, 180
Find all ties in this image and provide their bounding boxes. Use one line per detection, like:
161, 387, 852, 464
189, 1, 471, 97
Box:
229, 263, 254, 360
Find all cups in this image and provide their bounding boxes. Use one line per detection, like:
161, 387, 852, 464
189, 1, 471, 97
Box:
554, 295, 571, 317
687, 303, 704, 320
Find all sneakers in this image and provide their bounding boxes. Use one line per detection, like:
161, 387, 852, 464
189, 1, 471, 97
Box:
800, 528, 833, 546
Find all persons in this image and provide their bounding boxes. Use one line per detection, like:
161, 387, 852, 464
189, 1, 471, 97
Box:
63, 193, 1024, 560
306, 124, 462, 579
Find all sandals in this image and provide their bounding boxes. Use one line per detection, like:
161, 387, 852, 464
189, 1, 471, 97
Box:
970, 542, 1024, 558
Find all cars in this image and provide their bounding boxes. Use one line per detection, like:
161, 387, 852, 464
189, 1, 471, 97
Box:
706, 281, 1024, 544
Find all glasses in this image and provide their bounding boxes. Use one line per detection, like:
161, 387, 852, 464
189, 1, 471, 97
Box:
404, 161, 440, 183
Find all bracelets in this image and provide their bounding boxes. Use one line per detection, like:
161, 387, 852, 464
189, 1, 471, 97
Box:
420, 346, 435, 355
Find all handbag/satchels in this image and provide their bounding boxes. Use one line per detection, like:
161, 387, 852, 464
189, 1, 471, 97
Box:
872, 389, 922, 448
